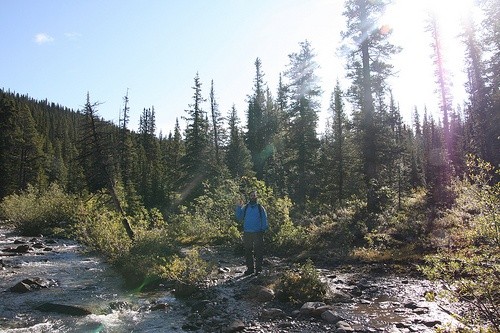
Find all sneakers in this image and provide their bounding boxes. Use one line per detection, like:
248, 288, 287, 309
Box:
244, 268, 262, 274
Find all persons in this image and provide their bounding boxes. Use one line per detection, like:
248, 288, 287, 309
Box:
237, 190, 268, 276
